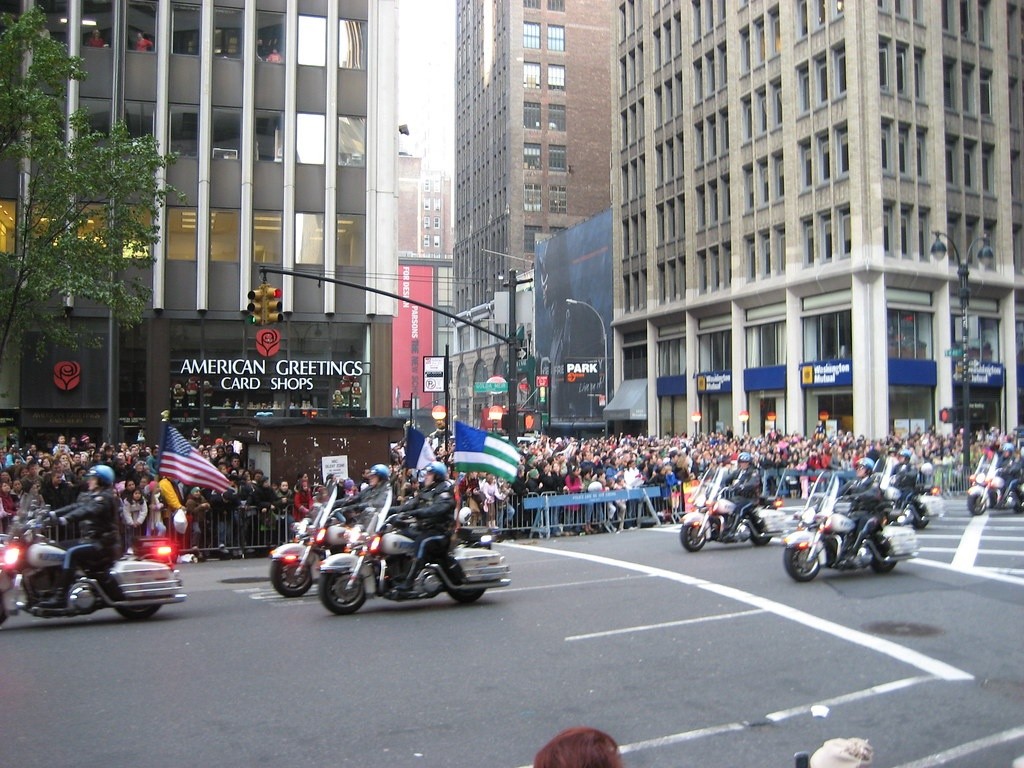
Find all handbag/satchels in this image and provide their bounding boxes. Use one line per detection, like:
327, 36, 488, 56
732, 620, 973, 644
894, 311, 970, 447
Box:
174, 509, 188, 534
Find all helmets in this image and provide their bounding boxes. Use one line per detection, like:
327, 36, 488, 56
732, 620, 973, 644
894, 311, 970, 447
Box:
82, 465, 115, 485
897, 449, 912, 459
738, 452, 753, 464
422, 461, 448, 476
366, 464, 392, 479
1002, 442, 1015, 452
856, 457, 875, 470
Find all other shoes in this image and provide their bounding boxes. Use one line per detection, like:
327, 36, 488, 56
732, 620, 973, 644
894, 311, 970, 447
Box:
585, 528, 598, 534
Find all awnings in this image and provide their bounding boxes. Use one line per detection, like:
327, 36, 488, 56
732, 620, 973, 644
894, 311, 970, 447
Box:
602, 378, 648, 420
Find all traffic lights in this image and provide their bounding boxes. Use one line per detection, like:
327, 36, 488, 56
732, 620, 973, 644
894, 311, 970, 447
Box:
248, 285, 263, 326
941, 407, 955, 424
263, 286, 285, 326
523, 412, 542, 432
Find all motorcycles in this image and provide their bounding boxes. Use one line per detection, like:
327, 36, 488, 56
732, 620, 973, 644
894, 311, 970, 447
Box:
268, 479, 515, 615
679, 465, 786, 552
780, 472, 920, 583
873, 456, 945, 532
966, 449, 1024, 516
0, 494, 190, 625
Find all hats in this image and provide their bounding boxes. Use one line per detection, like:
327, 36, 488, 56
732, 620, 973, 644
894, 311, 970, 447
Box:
191, 486, 201, 496
149, 481, 157, 492
809, 738, 874, 768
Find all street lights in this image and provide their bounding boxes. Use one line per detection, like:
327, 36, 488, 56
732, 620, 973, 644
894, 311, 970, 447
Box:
566, 299, 609, 439
929, 231, 994, 488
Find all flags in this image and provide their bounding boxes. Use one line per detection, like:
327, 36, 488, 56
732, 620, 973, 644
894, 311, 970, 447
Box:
454, 420, 521, 484
405, 426, 436, 469
154, 421, 232, 494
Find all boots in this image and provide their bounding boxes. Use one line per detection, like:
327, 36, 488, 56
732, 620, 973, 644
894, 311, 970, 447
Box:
35, 570, 75, 608
393, 559, 421, 590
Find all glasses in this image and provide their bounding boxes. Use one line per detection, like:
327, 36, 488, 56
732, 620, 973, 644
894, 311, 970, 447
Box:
370, 469, 378, 474
86, 469, 98, 476
425, 466, 434, 472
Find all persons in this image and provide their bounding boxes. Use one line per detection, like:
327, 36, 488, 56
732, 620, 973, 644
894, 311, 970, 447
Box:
313, 463, 390, 524
388, 461, 456, 592
995, 443, 1021, 509
533, 728, 624, 768
172, 375, 212, 407
813, 457, 879, 566
704, 452, 760, 540
36, 465, 123, 607
889, 449, 918, 516
810, 738, 874, 768
390, 419, 1024, 540
0, 434, 389, 561
332, 374, 362, 407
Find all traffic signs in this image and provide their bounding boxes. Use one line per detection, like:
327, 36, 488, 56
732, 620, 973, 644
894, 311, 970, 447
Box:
956, 357, 981, 367
516, 347, 528, 361
945, 349, 964, 357
475, 381, 508, 393
